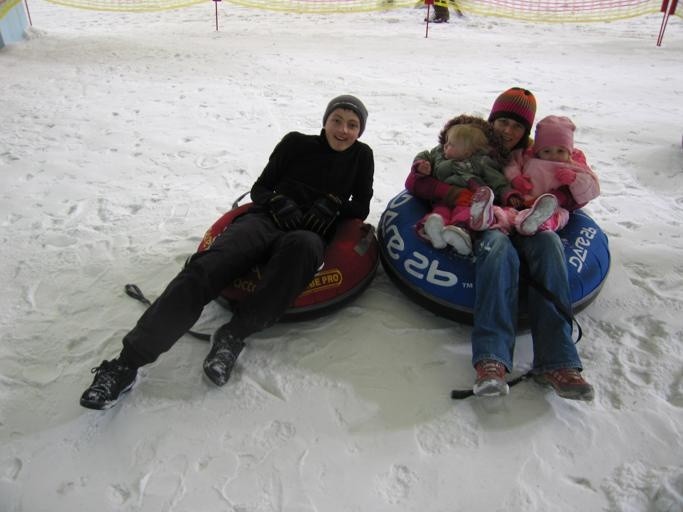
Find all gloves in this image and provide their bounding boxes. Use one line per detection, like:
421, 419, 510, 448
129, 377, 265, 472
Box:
300, 193, 344, 241
267, 194, 308, 231
511, 176, 533, 194
446, 185, 473, 206
557, 168, 576, 185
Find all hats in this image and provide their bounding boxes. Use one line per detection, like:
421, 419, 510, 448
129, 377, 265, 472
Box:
323, 94, 368, 138
533, 115, 576, 154
488, 86, 537, 131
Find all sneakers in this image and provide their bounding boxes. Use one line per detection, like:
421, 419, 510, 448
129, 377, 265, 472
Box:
424, 213, 447, 250
80, 358, 137, 410
472, 359, 510, 397
533, 367, 594, 401
202, 323, 247, 387
518, 192, 558, 237
469, 186, 494, 231
441, 224, 473, 256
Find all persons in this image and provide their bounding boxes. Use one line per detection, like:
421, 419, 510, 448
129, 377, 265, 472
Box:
401, 85, 596, 403
78, 93, 377, 412
421, 0, 454, 23
467, 114, 601, 241
410, 122, 524, 259
